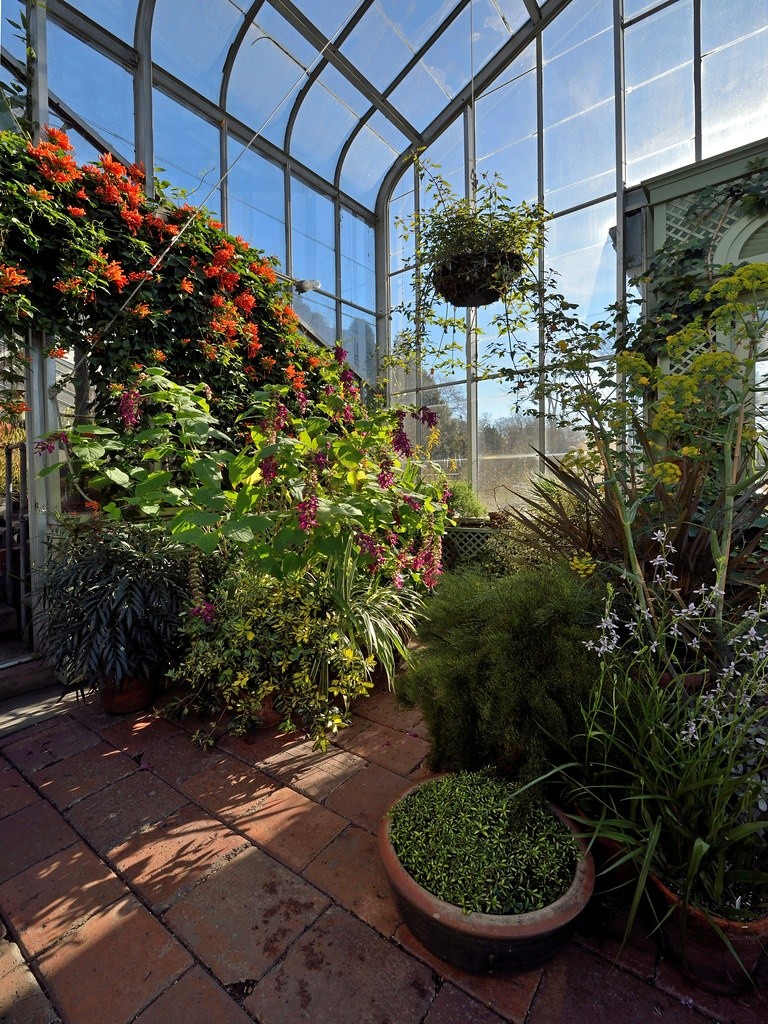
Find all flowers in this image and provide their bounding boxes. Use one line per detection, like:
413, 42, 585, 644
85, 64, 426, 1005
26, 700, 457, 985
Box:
486, 263, 768, 981
0, 121, 455, 754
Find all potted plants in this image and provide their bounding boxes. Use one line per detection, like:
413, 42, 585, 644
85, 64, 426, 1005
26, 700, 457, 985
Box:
377, 770, 600, 976
444, 478, 506, 575
375, 141, 602, 405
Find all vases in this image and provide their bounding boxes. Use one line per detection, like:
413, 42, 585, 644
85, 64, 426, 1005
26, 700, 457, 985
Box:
86, 657, 152, 718
625, 663, 713, 704
222, 683, 300, 731
613, 845, 768, 999
